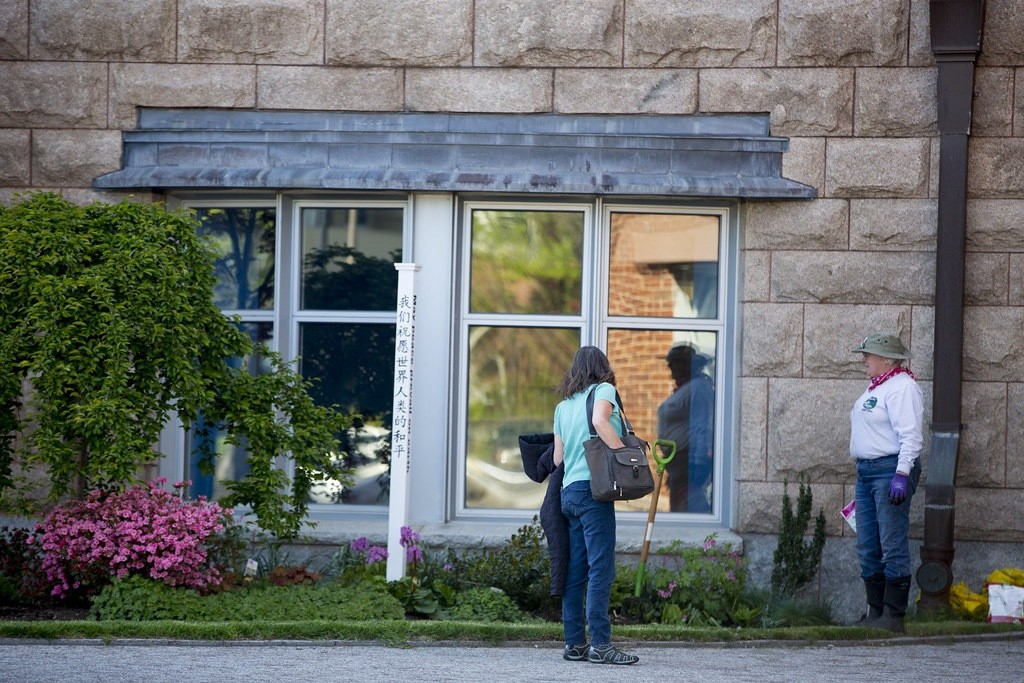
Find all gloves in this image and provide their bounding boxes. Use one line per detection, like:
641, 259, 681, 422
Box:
888, 473, 908, 505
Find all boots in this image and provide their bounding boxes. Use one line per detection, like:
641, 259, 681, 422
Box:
855, 572, 912, 633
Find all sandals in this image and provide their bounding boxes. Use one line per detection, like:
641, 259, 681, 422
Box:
588, 645, 638, 663
563, 644, 590, 661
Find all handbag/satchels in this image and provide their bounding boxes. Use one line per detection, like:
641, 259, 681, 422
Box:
582, 383, 655, 501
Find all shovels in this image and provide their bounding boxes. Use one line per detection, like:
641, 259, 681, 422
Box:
621, 437, 676, 623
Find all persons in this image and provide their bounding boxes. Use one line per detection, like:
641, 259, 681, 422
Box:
553, 346, 639, 665
850, 333, 923, 634
657, 346, 714, 513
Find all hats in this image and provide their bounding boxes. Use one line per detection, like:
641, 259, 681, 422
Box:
658, 344, 705, 362
851, 334, 914, 359
